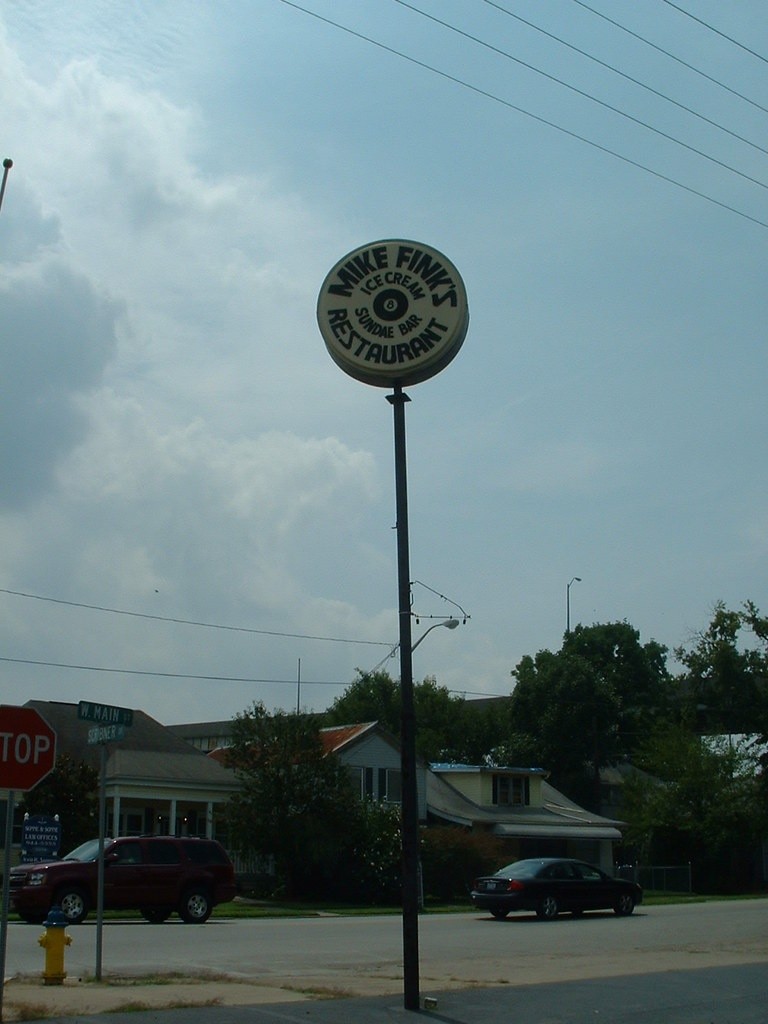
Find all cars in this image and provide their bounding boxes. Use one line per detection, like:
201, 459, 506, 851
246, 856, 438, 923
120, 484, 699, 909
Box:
468, 855, 643, 923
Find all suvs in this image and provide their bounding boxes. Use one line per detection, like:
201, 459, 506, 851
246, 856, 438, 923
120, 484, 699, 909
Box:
7, 832, 237, 926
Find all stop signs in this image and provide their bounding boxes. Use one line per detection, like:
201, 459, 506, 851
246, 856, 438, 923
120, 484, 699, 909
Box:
0, 703, 58, 792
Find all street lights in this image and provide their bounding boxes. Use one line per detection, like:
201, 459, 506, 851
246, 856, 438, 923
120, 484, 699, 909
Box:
566, 576, 583, 633
397, 618, 462, 1011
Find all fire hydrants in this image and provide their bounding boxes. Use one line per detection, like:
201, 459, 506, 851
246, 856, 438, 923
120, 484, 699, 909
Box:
35, 902, 73, 988
